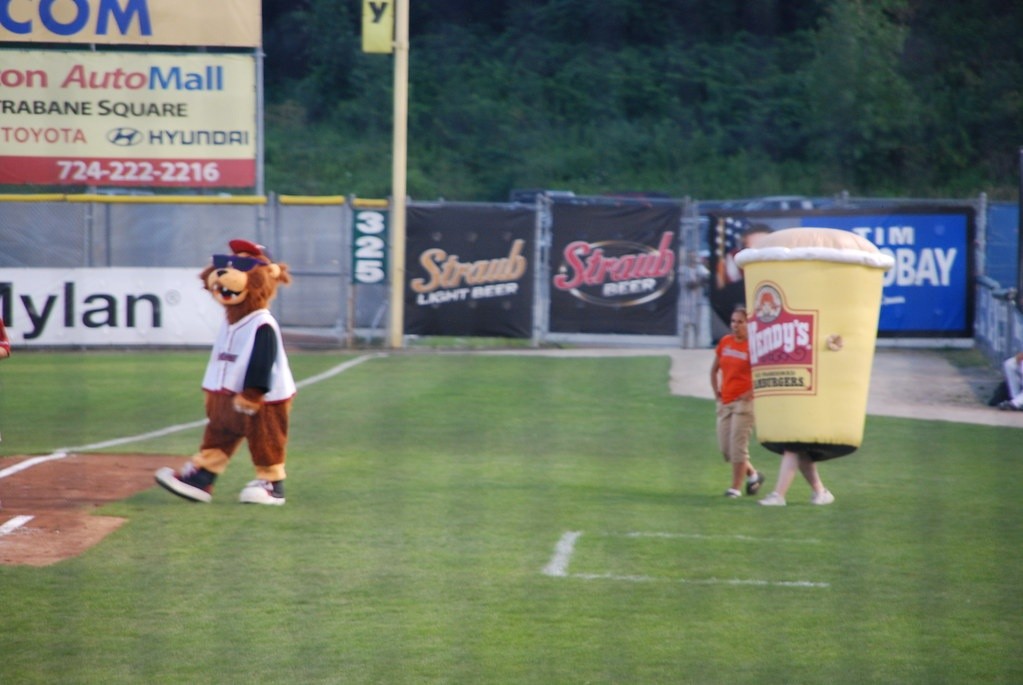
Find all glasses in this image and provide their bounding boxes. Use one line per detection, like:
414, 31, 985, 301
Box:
730, 318, 747, 324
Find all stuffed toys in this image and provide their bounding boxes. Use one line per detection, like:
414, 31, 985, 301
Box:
153, 239, 296, 507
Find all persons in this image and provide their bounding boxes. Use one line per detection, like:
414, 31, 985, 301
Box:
990, 353, 1023, 411
737, 226, 894, 505
707, 309, 762, 498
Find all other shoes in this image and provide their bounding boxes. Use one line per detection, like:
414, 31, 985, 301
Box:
758, 492, 785, 507
724, 491, 739, 499
811, 489, 835, 506
747, 472, 764, 496
